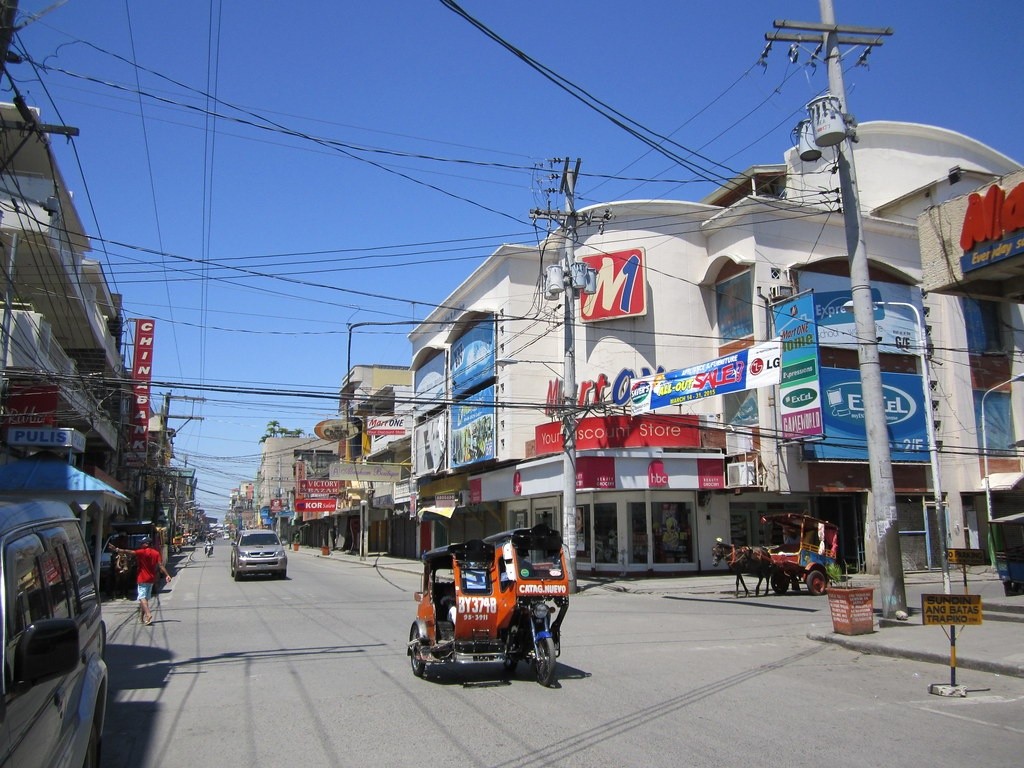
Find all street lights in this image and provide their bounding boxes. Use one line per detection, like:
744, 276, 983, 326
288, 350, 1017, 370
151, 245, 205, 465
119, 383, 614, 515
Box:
981, 373, 1024, 569
359, 500, 367, 562
841, 295, 953, 598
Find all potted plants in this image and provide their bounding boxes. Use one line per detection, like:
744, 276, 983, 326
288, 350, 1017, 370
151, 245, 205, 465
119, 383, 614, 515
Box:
823, 559, 876, 635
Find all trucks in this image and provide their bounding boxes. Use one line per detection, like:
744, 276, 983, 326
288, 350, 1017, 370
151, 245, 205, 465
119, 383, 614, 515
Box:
100, 520, 169, 585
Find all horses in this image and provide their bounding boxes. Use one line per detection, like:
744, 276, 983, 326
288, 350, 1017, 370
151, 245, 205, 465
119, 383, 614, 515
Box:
712, 542, 773, 598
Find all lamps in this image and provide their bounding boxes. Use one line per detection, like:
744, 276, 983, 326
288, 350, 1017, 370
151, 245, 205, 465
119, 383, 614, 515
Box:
947, 165, 1002, 186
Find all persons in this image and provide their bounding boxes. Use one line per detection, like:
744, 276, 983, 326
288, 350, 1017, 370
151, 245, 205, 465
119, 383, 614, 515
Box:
575, 508, 584, 537
108, 536, 172, 626
499, 544, 538, 597
204, 534, 214, 555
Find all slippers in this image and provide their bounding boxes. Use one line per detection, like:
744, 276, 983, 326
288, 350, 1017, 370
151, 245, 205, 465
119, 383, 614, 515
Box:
136, 619, 143, 624
145, 620, 153, 625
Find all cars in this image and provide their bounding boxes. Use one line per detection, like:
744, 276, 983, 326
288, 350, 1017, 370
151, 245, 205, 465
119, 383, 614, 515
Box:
1, 497, 109, 768
230, 529, 288, 581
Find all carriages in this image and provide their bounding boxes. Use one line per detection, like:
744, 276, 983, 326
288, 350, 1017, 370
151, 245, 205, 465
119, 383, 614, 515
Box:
712, 510, 840, 597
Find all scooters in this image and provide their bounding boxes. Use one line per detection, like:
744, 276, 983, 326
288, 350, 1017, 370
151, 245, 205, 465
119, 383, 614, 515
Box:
204, 542, 214, 557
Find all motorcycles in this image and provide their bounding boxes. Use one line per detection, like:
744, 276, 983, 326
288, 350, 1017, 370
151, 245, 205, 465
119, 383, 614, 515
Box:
407, 523, 570, 687
